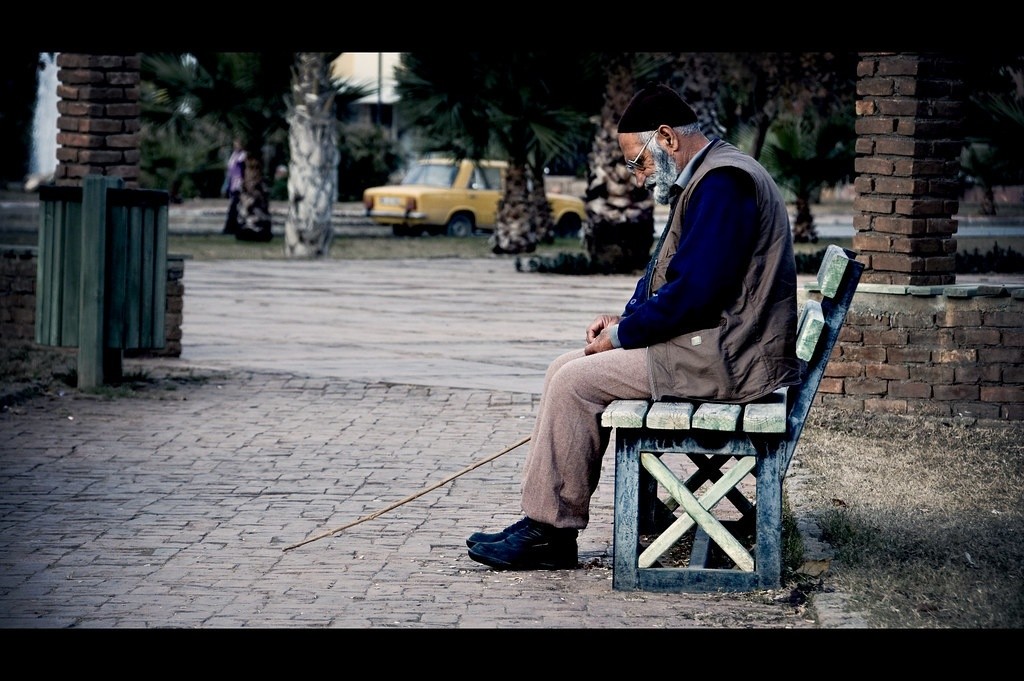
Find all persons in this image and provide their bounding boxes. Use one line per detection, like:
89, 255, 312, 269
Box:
466, 85, 802, 571
221, 139, 247, 235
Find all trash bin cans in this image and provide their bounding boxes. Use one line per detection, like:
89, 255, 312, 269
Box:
34, 179, 171, 387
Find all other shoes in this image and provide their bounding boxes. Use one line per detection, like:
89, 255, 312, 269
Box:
466, 516, 578, 570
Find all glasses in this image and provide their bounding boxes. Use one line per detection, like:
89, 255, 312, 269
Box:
626, 130, 658, 175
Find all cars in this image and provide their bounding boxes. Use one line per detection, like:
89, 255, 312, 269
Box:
364, 159, 587, 240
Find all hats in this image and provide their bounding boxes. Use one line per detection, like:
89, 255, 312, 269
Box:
617, 81, 698, 133
